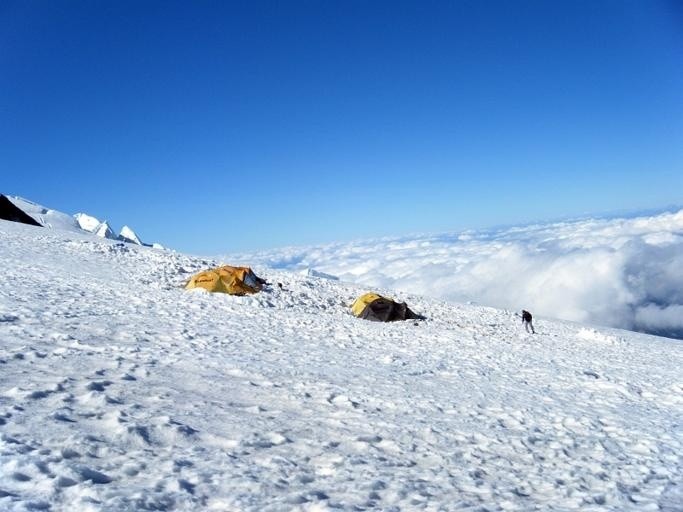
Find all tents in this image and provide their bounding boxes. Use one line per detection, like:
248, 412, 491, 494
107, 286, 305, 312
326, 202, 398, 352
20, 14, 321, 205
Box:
363, 300, 427, 322
350, 292, 393, 317
186, 265, 266, 295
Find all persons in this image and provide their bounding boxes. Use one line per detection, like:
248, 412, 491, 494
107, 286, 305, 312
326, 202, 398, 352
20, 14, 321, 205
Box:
521, 310, 535, 334
